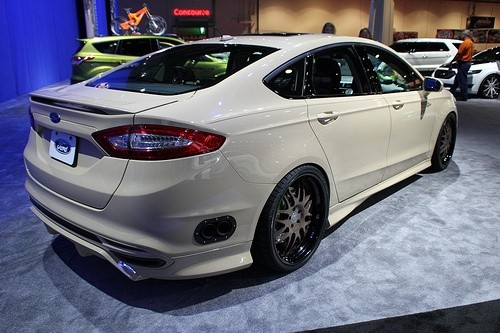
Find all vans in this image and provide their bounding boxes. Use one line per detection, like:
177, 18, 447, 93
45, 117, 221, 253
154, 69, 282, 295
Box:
70, 34, 182, 91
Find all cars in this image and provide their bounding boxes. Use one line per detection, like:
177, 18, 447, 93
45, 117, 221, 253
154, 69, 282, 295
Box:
432, 47, 500, 100
385, 38, 469, 71
24, 31, 459, 284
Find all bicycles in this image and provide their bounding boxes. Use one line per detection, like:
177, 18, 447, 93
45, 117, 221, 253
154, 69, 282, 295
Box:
110, 2, 168, 35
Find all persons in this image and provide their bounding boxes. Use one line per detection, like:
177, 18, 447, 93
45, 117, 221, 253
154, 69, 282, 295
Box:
358, 28, 372, 39
446, 29, 474, 101
321, 23, 336, 34
